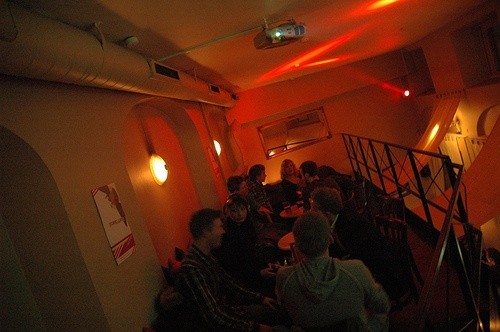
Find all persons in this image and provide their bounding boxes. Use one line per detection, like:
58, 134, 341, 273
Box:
474, 247, 500, 332
151, 158, 413, 331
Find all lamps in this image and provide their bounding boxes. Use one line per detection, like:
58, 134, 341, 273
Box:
149, 153, 168, 186
213, 139, 222, 158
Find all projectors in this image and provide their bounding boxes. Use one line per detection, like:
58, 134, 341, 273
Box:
254, 23, 307, 49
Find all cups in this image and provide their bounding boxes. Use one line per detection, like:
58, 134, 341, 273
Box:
282, 200, 293, 215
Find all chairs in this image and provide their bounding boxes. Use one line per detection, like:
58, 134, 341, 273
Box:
156, 248, 199, 332
289, 242, 305, 265
342, 173, 424, 312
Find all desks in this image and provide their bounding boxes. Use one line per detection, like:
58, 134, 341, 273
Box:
278, 231, 297, 250
280, 204, 304, 229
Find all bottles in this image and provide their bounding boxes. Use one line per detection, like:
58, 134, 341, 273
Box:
290, 243, 304, 266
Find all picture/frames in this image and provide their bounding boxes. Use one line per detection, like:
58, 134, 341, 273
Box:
258, 105, 334, 160
